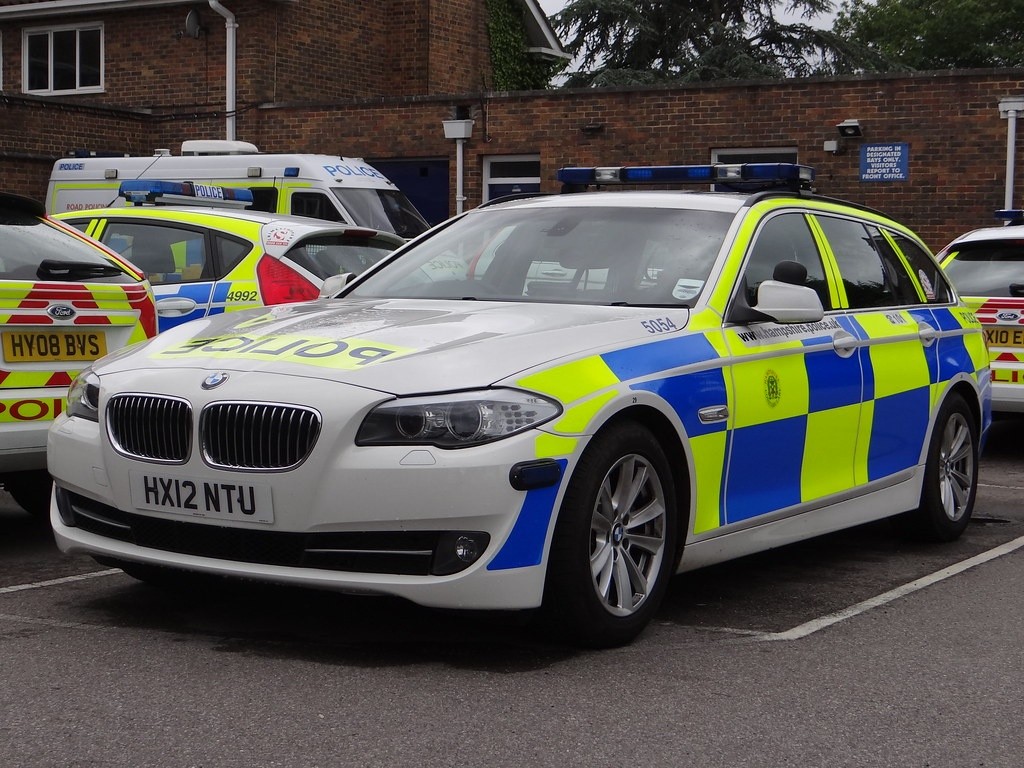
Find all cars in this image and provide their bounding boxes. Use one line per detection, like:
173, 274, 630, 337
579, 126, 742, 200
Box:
50, 179, 413, 335
0, 193, 157, 513
931, 206, 1024, 419
44, 160, 992, 651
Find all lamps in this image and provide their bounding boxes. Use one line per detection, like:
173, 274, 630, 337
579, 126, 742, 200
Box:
580, 122, 605, 133
837, 118, 864, 139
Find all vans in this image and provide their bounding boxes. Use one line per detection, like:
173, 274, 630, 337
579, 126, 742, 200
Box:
43, 139, 469, 284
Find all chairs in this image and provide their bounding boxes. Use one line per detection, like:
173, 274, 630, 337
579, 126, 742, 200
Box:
127, 234, 175, 283
558, 236, 626, 302
